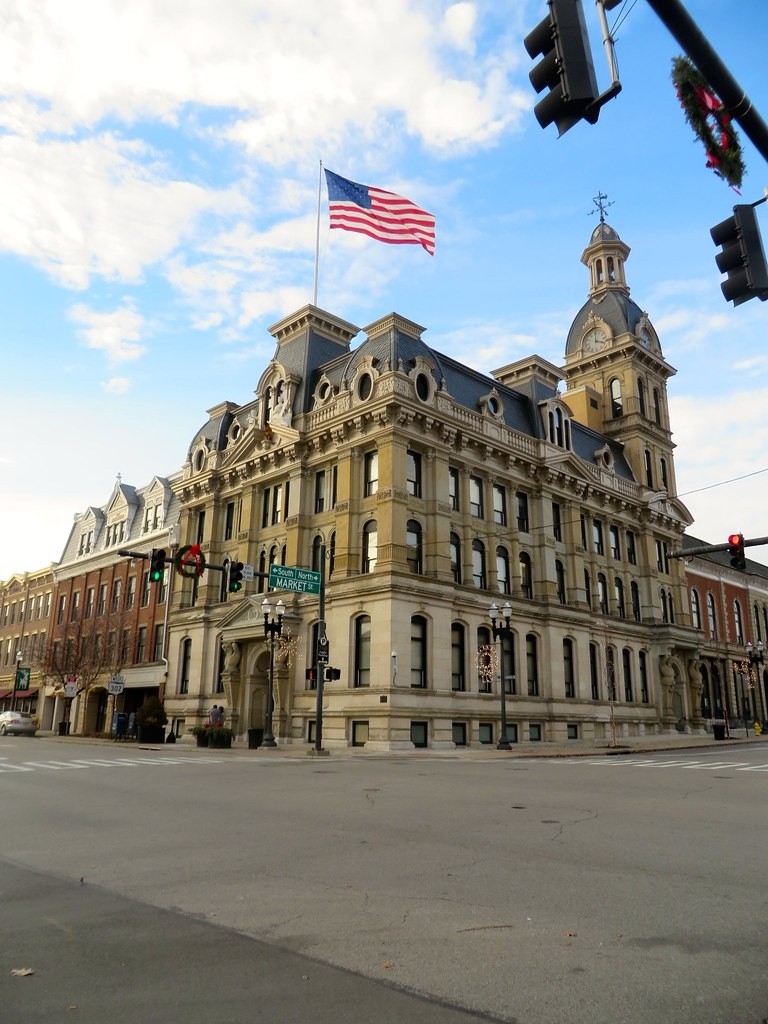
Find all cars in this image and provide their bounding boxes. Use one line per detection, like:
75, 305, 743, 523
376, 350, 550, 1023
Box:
0, 711, 37, 737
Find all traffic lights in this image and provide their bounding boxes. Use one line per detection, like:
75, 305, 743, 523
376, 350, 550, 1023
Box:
147, 548, 168, 583
728, 533, 747, 570
305, 668, 317, 681
523, 0, 603, 141
228, 560, 244, 593
710, 204, 768, 307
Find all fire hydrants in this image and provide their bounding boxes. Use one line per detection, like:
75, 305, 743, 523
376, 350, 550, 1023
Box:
753, 722, 762, 736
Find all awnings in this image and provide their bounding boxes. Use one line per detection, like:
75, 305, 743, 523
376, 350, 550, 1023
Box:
0, 691, 12, 699
6, 688, 39, 698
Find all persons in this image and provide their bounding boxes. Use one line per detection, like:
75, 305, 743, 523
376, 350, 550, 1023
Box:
273, 397, 284, 421
208, 705, 226, 728
246, 409, 259, 429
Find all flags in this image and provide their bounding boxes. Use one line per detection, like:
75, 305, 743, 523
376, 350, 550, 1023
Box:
324, 168, 436, 255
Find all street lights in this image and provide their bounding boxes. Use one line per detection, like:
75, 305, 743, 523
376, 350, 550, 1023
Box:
745, 640, 768, 735
9, 650, 25, 712
488, 600, 513, 750
260, 598, 286, 747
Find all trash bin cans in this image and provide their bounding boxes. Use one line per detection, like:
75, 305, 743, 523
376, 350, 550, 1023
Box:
248, 728, 263, 749
111, 713, 128, 740
59, 722, 71, 736
712, 725, 725, 740
